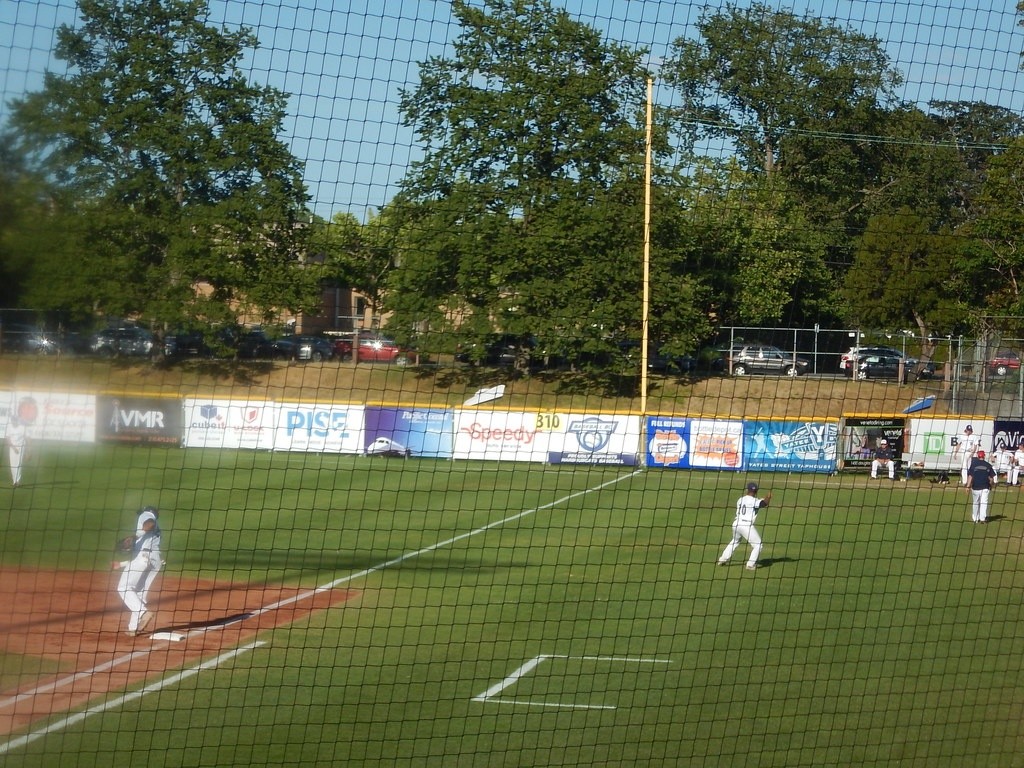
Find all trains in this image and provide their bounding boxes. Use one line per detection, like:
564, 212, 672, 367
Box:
368, 438, 407, 457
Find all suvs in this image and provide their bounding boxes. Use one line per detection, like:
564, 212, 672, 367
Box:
725, 345, 810, 377
839, 345, 908, 369
989, 349, 1022, 376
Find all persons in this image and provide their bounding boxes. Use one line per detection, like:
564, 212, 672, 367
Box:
954, 425, 978, 486
965, 451, 996, 524
718, 482, 773, 571
870, 440, 895, 480
990, 444, 1015, 486
108, 506, 166, 636
1012, 443, 1024, 486
5, 419, 27, 487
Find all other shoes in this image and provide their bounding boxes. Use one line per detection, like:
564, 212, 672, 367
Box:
870, 477, 876, 480
975, 520, 987, 524
746, 566, 757, 570
718, 561, 727, 566
889, 478, 895, 480
1013, 484, 1016, 486
963, 484, 966, 487
994, 483, 997, 487
1006, 483, 1011, 486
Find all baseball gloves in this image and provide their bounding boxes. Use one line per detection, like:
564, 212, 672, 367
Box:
116, 534, 138, 555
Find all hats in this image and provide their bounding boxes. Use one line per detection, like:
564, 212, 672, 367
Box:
880, 439, 887, 445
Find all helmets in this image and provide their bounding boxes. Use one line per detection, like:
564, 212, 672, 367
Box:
1000, 443, 1007, 448
966, 425, 972, 431
978, 451, 984, 458
748, 483, 758, 492
140, 506, 159, 519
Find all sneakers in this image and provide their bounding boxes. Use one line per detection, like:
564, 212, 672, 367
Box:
137, 610, 153, 631
124, 630, 140, 636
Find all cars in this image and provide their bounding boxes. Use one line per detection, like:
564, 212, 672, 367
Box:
846, 354, 934, 380
89, 326, 172, 358
0, 324, 60, 356
169, 322, 335, 363
337, 333, 430, 368
456, 335, 554, 366
